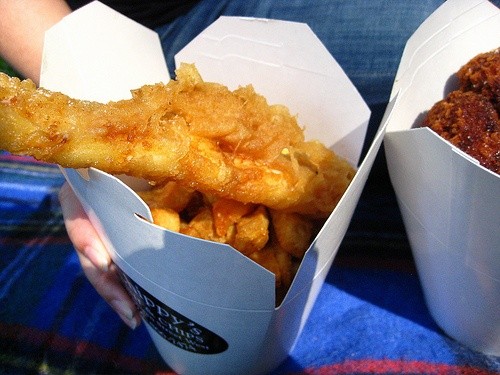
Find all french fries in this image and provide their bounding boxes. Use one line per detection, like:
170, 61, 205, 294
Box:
131, 182, 314, 306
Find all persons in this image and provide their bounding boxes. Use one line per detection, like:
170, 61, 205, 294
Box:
0, 1, 147, 330
